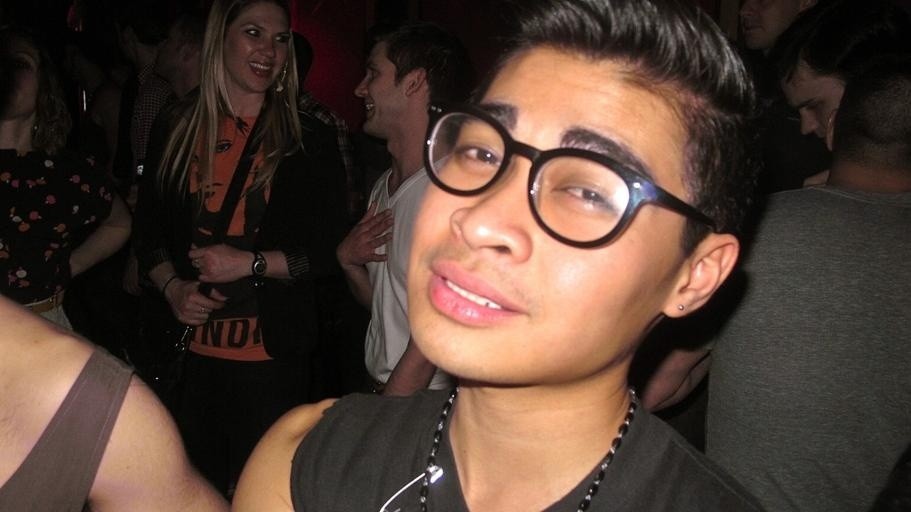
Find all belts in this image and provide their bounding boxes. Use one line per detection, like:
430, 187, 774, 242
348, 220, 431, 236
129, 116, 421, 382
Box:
29, 292, 65, 315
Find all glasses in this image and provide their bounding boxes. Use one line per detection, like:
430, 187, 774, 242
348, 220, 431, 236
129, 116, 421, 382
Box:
422, 99, 716, 251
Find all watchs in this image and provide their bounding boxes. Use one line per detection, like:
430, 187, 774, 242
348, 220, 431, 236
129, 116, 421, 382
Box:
252, 253, 266, 279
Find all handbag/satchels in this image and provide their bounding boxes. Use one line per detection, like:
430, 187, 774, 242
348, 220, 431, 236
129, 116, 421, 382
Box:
106, 297, 185, 393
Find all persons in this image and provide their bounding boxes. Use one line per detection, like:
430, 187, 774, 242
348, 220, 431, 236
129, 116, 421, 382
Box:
0, 0, 208, 330
635, 68, 909, 511
736, 0, 833, 193
230, 0, 781, 511
767, 0, 909, 190
335, 9, 475, 397
0, 294, 229, 511
291, 33, 362, 215
132, 1, 348, 497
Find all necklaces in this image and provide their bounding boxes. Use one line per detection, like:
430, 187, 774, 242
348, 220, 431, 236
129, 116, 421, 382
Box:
419, 386, 636, 511
